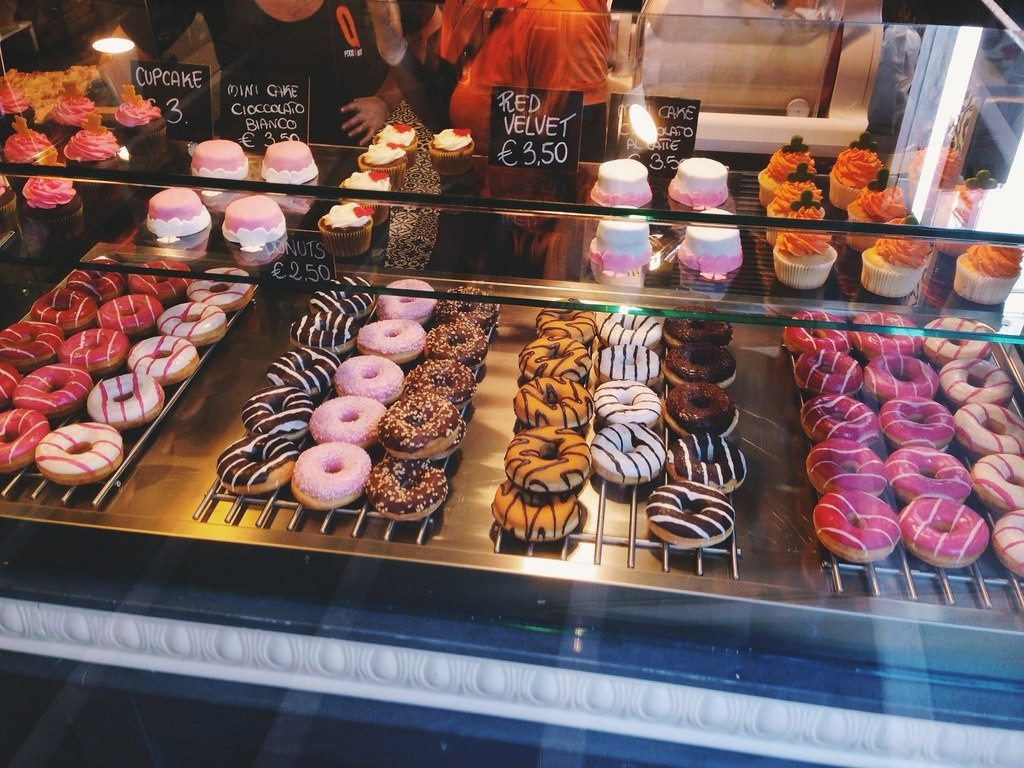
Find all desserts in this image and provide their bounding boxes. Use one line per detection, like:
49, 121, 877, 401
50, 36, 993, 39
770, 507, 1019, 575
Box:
594, 135, 1022, 304
0, 83, 474, 266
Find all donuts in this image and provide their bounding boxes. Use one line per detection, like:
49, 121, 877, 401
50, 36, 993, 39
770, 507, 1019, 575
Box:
0, 259, 1024, 592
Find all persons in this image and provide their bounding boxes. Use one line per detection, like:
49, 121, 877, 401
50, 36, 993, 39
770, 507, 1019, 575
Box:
96, 0, 610, 280
